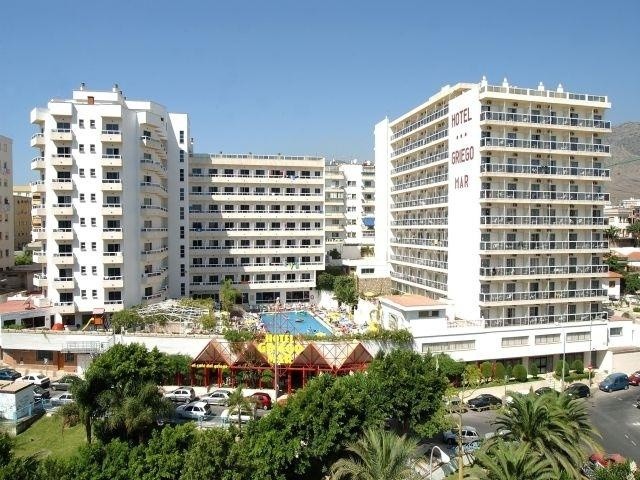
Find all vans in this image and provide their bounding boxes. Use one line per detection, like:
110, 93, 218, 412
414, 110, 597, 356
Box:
176, 402, 211, 421
165, 387, 195, 404
248, 392, 271, 410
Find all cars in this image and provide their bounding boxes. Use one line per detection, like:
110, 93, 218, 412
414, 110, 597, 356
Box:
564, 382, 590, 400
51, 380, 73, 390
536, 386, 559, 399
50, 392, 76, 405
31, 385, 50, 402
599, 373, 629, 393
443, 398, 468, 414
446, 428, 479, 445
506, 391, 531, 405
14, 373, 50, 388
630, 370, 640, 387
0, 369, 21, 381
201, 391, 232, 407
220, 408, 254, 424
469, 395, 501, 412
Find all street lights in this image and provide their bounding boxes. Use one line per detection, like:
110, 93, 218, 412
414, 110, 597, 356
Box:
429, 446, 450, 480
